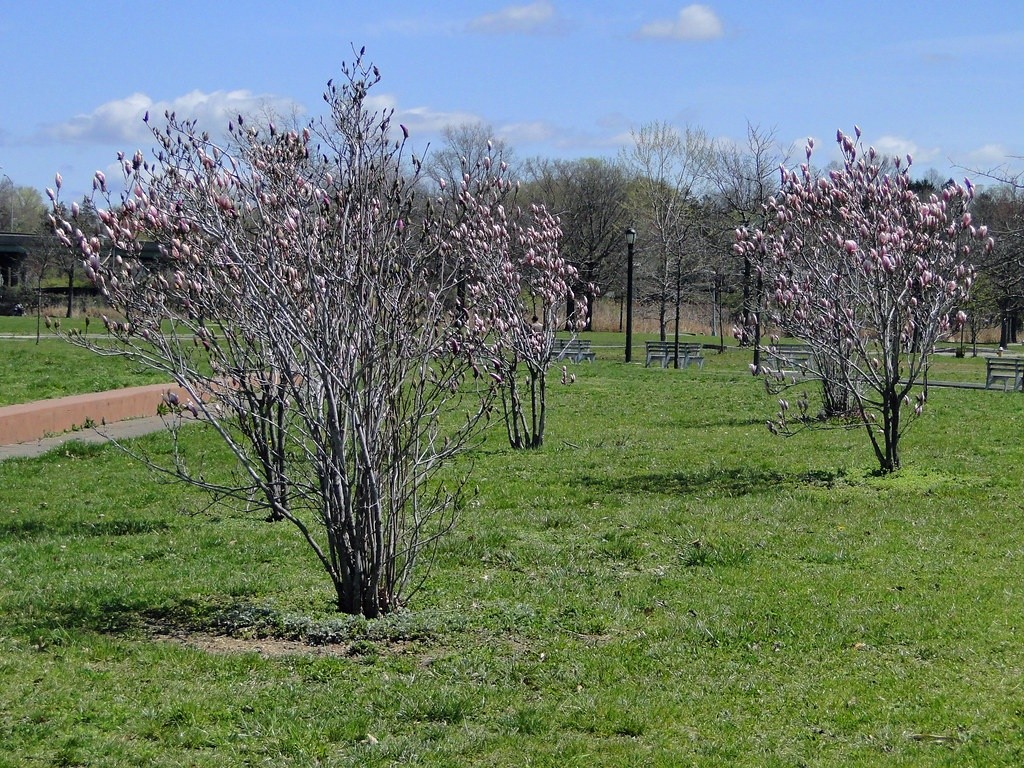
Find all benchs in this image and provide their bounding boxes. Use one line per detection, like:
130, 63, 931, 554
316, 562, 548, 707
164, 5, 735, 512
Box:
550, 339, 596, 363
767, 343, 814, 372
645, 340, 705, 370
985, 357, 1024, 391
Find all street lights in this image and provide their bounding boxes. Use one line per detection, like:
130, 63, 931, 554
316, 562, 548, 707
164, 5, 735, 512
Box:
623, 225, 637, 362
